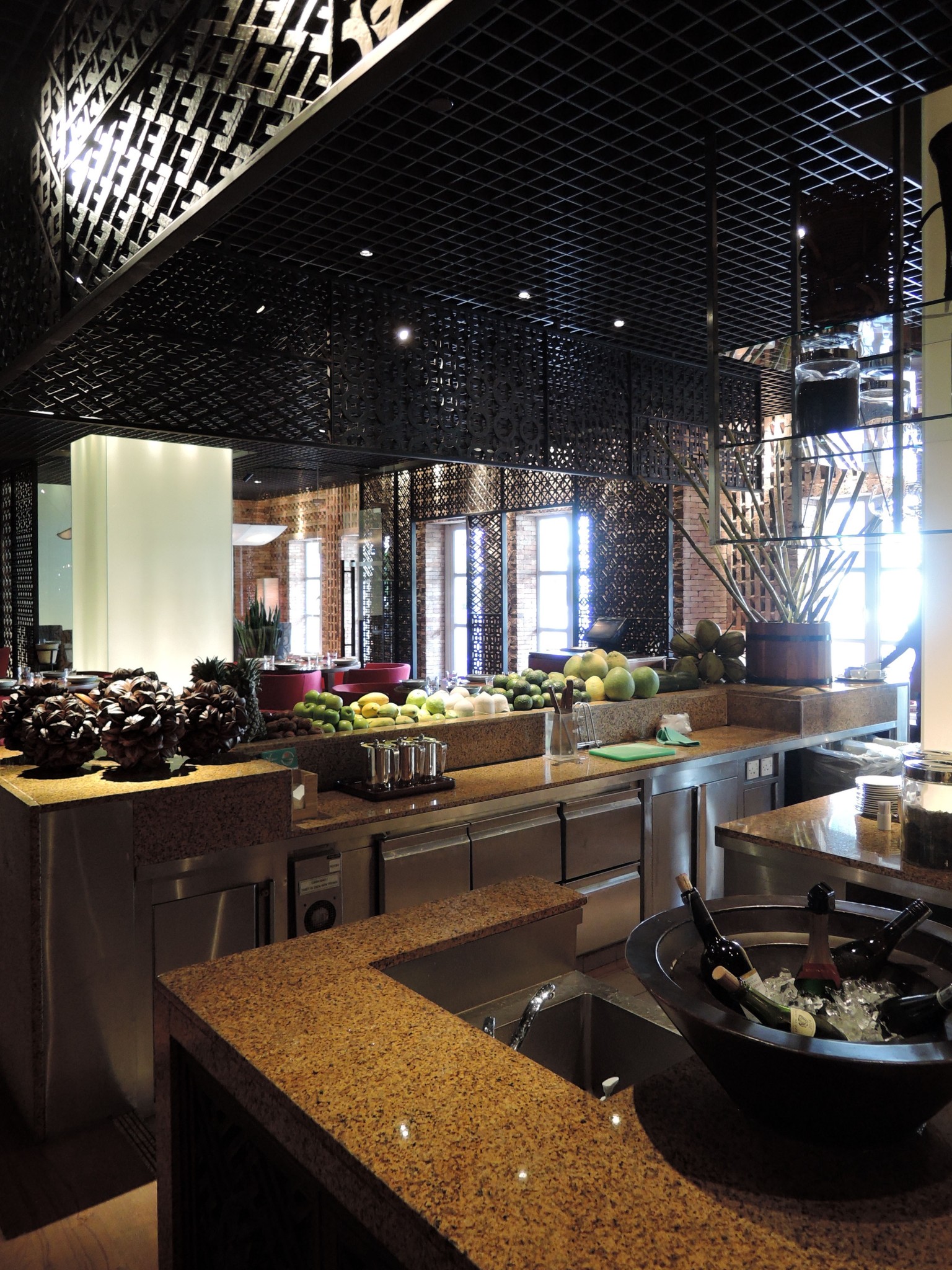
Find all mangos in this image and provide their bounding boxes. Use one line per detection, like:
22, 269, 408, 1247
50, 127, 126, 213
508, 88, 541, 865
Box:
350, 692, 418, 727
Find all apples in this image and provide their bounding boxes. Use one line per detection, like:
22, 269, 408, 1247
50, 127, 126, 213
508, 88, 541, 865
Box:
293, 689, 369, 733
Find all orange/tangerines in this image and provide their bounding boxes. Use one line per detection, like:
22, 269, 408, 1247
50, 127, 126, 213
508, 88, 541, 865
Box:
469, 668, 591, 712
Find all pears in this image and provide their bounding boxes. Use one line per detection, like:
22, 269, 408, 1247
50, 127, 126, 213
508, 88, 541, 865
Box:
404, 689, 459, 722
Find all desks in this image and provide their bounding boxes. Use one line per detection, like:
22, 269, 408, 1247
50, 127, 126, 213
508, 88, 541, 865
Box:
255, 659, 361, 693
395, 678, 487, 695
714, 780, 952, 908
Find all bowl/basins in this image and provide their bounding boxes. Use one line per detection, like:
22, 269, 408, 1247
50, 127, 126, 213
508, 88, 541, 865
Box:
624, 893, 952, 1142
0, 679, 17, 686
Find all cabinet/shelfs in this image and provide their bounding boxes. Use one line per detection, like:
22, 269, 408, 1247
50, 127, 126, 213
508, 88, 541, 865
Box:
528, 649, 668, 675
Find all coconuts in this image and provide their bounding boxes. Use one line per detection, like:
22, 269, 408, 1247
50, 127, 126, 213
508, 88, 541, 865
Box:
670, 620, 746, 683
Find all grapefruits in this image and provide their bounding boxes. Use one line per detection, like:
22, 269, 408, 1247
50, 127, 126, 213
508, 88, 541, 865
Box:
548, 649, 660, 701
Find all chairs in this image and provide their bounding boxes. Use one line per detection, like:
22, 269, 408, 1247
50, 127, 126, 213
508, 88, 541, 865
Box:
255, 663, 411, 714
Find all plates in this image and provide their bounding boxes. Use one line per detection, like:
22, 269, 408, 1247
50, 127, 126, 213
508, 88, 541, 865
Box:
273, 661, 301, 668
65, 675, 98, 684
246, 657, 271, 663
835, 676, 885, 681
854, 775, 901, 817
399, 673, 496, 694
40, 670, 72, 678
300, 655, 325, 661
331, 658, 358, 666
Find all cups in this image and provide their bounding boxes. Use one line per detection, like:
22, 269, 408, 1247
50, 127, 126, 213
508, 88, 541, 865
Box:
17, 666, 43, 687
426, 670, 494, 695
64, 668, 77, 683
877, 812, 891, 831
850, 662, 881, 679
890, 794, 901, 823
264, 650, 337, 670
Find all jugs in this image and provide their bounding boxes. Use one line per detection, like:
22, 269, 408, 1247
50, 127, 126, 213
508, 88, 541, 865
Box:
358, 732, 447, 791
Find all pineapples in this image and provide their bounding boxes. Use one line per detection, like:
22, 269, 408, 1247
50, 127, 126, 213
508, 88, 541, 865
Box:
190, 653, 264, 734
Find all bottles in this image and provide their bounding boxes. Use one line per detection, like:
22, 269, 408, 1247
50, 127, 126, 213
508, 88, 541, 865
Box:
897, 747, 951, 870
674, 870, 952, 1041
